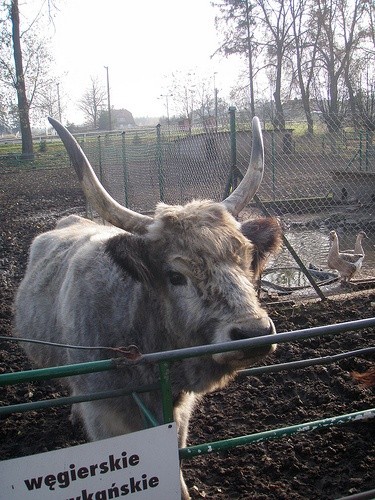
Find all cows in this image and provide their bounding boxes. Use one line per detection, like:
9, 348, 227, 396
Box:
13, 116, 283, 500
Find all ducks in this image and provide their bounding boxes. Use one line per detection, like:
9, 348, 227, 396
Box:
327, 231, 364, 287
338, 230, 368, 257
